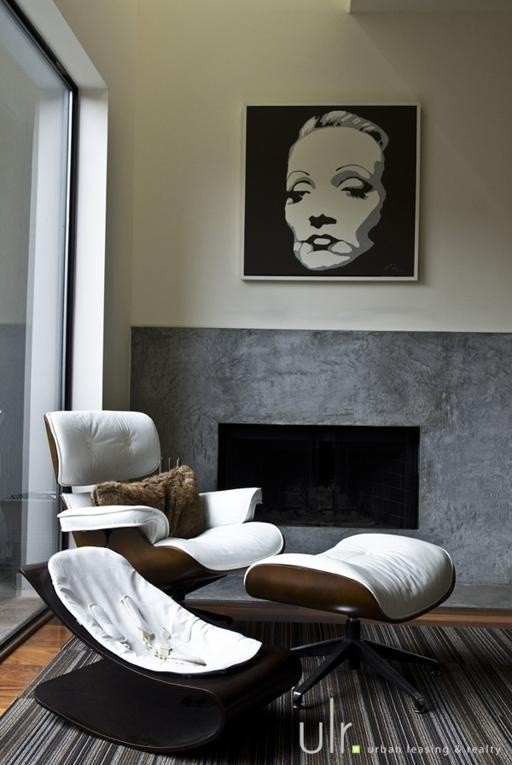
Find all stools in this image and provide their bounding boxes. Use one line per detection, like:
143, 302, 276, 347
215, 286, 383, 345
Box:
239, 529, 458, 716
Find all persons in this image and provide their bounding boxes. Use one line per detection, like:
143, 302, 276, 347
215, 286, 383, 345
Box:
280, 110, 392, 275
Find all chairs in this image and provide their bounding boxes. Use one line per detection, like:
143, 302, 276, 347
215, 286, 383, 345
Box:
41, 408, 286, 630
14, 543, 305, 757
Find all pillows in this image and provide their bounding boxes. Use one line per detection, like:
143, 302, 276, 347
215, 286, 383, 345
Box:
90, 462, 207, 540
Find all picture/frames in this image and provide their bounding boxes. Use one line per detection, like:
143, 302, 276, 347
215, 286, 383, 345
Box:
237, 98, 425, 288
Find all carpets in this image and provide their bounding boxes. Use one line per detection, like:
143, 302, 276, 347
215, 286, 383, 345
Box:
0, 612, 512, 765
